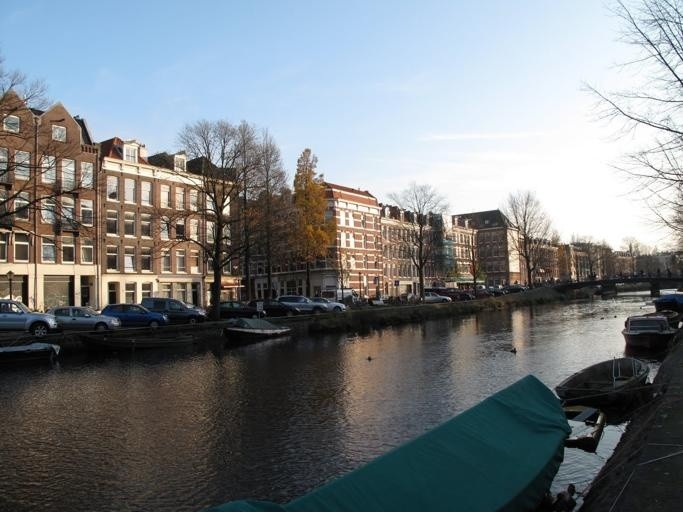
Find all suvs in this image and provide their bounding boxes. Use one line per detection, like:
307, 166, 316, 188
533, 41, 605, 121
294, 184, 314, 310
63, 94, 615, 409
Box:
141, 297, 206, 324
276, 295, 328, 315
0, 298, 58, 337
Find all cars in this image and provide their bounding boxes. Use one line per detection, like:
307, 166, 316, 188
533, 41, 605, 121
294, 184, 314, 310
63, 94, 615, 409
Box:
48, 306, 121, 332
246, 298, 301, 317
205, 300, 267, 321
424, 283, 524, 304
310, 297, 347, 312
100, 303, 169, 327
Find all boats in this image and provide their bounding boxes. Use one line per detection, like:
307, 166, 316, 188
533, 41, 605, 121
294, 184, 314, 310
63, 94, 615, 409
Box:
556, 310, 680, 447
0, 337, 60, 364
222, 318, 290, 339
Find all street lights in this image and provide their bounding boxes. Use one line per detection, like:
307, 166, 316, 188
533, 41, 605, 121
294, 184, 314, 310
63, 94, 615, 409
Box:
7, 271, 14, 310
